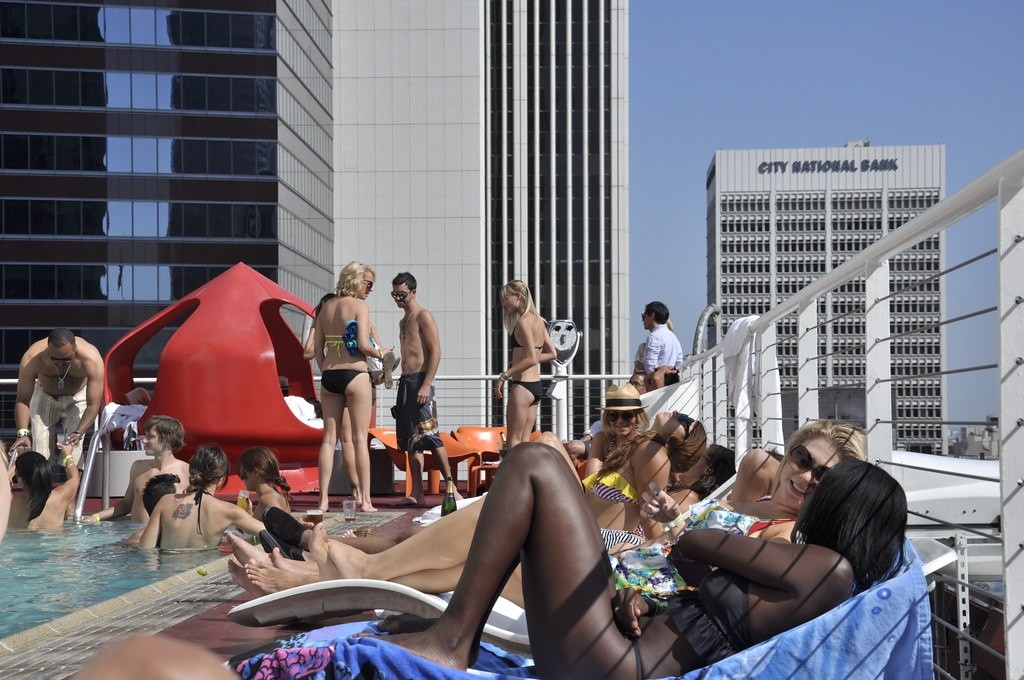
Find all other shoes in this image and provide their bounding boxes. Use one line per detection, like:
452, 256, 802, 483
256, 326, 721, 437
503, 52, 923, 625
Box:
259, 529, 307, 565
262, 505, 310, 550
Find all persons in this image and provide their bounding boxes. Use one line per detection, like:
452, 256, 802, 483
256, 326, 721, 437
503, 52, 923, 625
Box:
226, 261, 867, 610
138, 443, 269, 549
82, 415, 191, 524
126, 474, 187, 543
238, 446, 294, 538
352, 443, 908, 680
391, 272, 466, 505
0, 328, 103, 542
494, 280, 558, 450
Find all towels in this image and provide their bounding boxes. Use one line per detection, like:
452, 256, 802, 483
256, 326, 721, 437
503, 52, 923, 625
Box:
722, 314, 786, 473
226, 540, 936, 680
283, 395, 324, 429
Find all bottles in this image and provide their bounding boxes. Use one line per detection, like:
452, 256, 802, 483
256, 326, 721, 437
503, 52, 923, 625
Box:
441, 477, 457, 517
499, 431, 507, 458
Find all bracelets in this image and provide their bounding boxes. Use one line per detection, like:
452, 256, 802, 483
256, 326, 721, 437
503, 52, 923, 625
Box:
92, 514, 100, 523
16, 429, 30, 434
65, 463, 75, 473
501, 371, 508, 379
62, 456, 73, 467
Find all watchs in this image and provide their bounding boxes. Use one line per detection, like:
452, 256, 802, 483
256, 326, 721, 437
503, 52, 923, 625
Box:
17, 431, 30, 438
73, 431, 83, 439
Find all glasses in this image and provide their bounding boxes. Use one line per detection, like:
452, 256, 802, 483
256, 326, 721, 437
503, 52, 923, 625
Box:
390, 290, 411, 298
642, 313, 648, 319
677, 413, 694, 441
608, 411, 636, 423
647, 379, 652, 386
627, 381, 645, 388
788, 445, 830, 482
51, 349, 74, 364
361, 280, 373, 291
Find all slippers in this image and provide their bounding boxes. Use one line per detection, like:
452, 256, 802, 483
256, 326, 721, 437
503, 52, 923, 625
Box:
375, 352, 401, 390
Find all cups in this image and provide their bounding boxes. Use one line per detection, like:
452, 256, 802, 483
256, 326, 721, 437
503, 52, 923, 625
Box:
238, 490, 249, 512
307, 509, 323, 530
134, 436, 146, 451
496, 442, 506, 462
343, 500, 356, 521
57, 433, 69, 449
16, 447, 31, 487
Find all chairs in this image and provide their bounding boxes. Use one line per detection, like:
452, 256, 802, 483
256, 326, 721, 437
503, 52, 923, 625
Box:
226, 534, 962, 680
367, 426, 544, 504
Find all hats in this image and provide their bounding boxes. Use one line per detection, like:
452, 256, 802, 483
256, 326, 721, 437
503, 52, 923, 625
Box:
595, 384, 648, 410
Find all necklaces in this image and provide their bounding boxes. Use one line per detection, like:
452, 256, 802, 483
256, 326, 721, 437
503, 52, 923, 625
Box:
55, 363, 72, 389
401, 311, 412, 343
